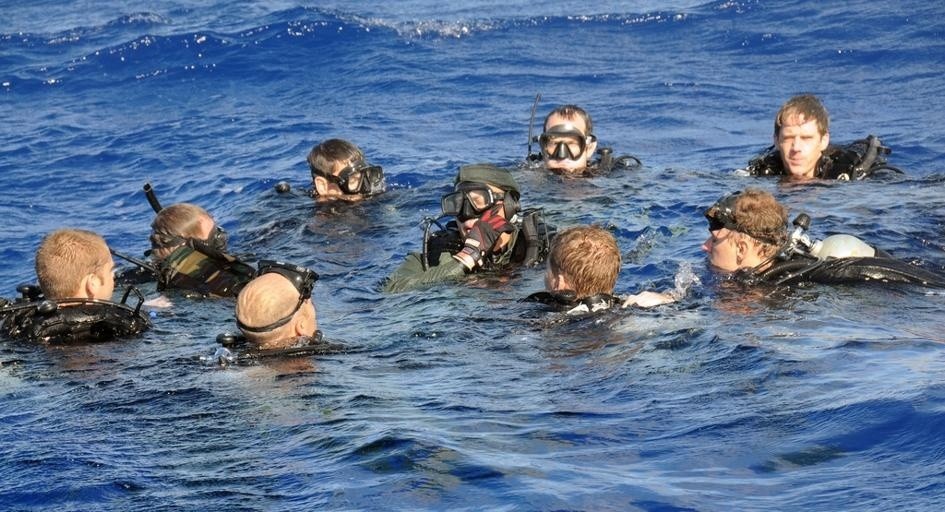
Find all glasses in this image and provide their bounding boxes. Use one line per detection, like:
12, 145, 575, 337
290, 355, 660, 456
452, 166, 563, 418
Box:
534, 125, 591, 159
704, 191, 780, 248
314, 160, 387, 198
441, 185, 506, 222
251, 258, 319, 300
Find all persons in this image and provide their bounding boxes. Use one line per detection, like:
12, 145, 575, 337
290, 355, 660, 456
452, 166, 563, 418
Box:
111, 203, 259, 300
375, 164, 559, 293
304, 136, 374, 202
211, 259, 343, 368
698, 186, 945, 287
517, 223, 673, 314
501, 103, 643, 183
5, 227, 154, 349
747, 93, 890, 182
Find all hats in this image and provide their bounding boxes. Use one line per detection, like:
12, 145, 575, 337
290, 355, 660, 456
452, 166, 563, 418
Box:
455, 163, 521, 199
